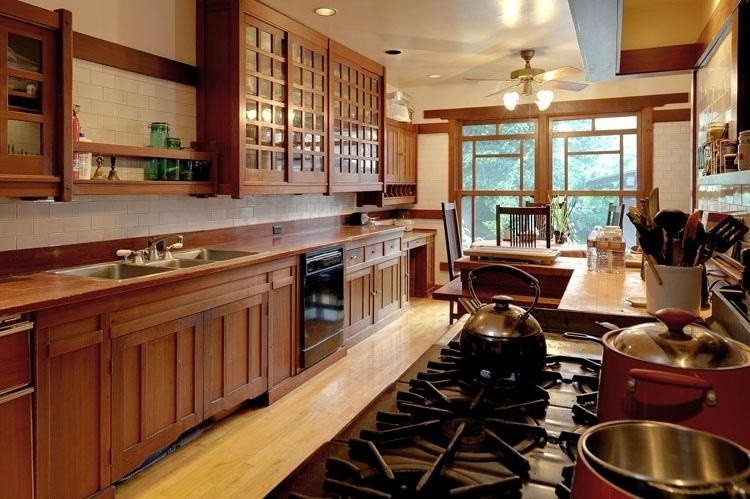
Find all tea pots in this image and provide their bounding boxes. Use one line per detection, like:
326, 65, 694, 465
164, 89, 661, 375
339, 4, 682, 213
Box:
459, 263, 547, 375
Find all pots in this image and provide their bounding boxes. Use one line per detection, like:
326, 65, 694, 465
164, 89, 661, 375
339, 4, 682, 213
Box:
571, 419, 750, 499
562, 308, 750, 450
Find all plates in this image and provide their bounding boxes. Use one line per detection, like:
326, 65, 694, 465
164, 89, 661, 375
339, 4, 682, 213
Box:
626, 296, 647, 307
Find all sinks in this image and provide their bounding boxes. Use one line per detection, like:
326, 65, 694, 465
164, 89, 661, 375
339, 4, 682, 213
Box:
42, 260, 175, 282
170, 247, 259, 264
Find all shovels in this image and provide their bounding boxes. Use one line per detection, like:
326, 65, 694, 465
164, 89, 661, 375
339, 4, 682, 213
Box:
638, 225, 666, 265
694, 216, 750, 266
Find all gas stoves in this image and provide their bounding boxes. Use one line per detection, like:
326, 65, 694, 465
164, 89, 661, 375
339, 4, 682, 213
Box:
259, 343, 602, 499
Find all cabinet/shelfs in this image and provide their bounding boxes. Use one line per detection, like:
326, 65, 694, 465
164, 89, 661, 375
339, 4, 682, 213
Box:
1, 0, 74, 207
4, 313, 34, 497
404, 236, 435, 304
195, 0, 328, 200
355, 118, 419, 208
328, 38, 388, 198
106, 275, 271, 487
33, 296, 106, 498
344, 237, 404, 342
74, 138, 219, 200
268, 266, 297, 389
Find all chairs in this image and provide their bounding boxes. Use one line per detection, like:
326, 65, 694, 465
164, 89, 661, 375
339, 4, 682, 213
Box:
525, 201, 565, 240
494, 203, 551, 250
440, 200, 465, 326
606, 202, 625, 227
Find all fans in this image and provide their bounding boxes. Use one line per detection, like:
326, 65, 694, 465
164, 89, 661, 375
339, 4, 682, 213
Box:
461, 49, 590, 99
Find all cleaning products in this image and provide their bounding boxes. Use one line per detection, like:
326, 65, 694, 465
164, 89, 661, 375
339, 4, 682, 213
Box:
79, 125, 93, 180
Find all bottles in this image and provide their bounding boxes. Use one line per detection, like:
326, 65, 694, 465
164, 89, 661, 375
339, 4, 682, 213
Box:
596, 225, 625, 275
147, 122, 199, 181
586, 226, 602, 270
503, 228, 511, 241
80, 133, 92, 180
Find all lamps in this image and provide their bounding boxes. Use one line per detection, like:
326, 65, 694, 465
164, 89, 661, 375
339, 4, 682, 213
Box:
502, 88, 554, 112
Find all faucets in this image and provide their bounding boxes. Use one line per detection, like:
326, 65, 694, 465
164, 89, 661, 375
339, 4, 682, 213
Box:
142, 234, 186, 261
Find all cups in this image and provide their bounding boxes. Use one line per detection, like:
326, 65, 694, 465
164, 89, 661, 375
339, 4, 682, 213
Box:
643, 260, 703, 316
